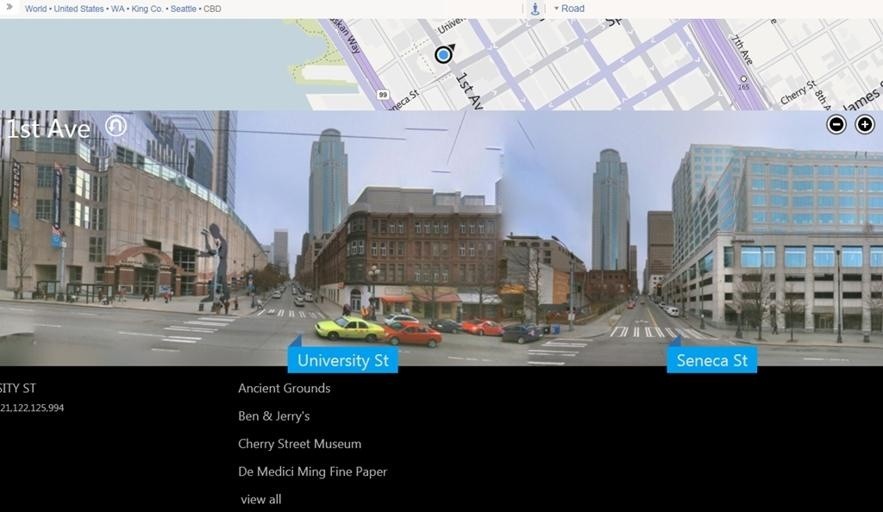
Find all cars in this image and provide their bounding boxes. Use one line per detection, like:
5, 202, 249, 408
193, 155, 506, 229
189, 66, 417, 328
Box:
314, 312, 543, 346
627, 295, 679, 316
273, 283, 313, 307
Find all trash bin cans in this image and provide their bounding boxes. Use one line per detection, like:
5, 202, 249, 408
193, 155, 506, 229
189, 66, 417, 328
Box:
199, 304, 203, 311
543, 325, 560, 335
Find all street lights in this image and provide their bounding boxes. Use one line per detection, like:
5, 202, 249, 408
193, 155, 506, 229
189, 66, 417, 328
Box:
368, 266, 381, 319
552, 235, 574, 330
252, 250, 269, 308
835, 243, 842, 343
698, 268, 708, 329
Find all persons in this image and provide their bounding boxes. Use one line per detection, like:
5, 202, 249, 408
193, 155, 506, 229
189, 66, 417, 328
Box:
341, 303, 374, 320
214, 295, 239, 315
36, 283, 175, 306
